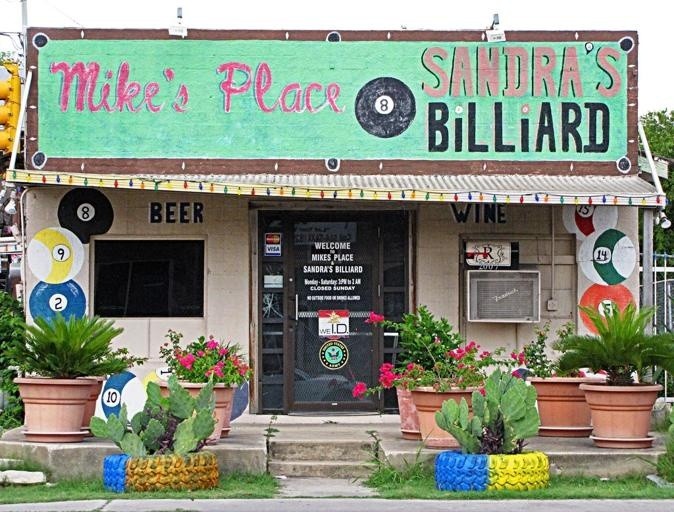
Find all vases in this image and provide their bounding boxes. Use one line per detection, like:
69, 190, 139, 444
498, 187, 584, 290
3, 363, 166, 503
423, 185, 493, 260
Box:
150, 382, 238, 444
394, 378, 481, 448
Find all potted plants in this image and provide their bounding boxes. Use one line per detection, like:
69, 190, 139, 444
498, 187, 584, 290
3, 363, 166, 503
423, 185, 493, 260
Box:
3, 313, 149, 442
524, 300, 674, 449
435, 369, 550, 492
89, 375, 218, 491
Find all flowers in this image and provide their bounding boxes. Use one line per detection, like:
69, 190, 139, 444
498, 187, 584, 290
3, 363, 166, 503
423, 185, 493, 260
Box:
158, 328, 255, 389
353, 304, 530, 402
